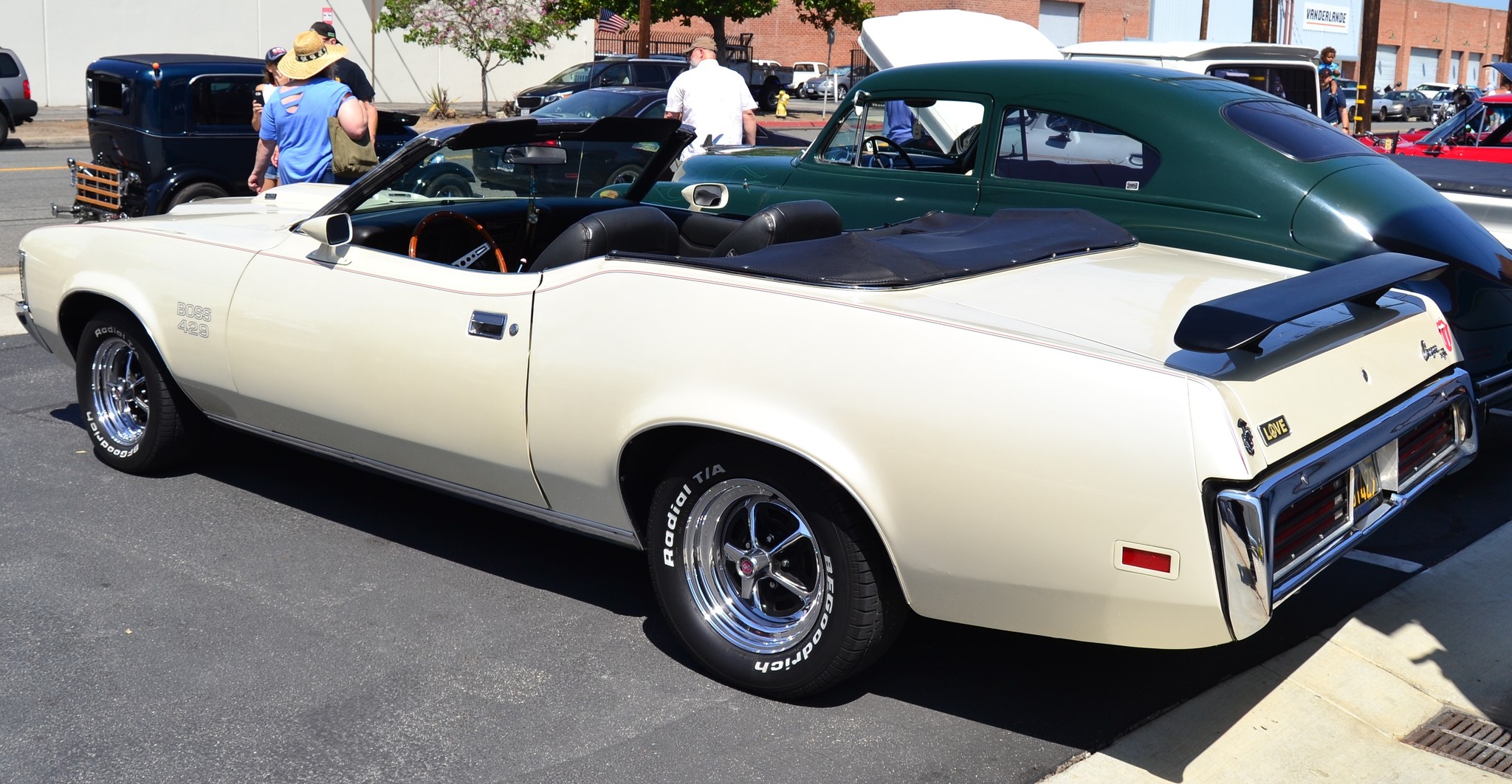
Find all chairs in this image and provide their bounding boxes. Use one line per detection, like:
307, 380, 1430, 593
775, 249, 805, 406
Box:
528, 206, 679, 273
710, 199, 842, 258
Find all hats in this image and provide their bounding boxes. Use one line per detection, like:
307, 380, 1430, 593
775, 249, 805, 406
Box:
265, 47, 287, 63
682, 35, 718, 54
1319, 68, 1332, 77
276, 30, 348, 80
1502, 76, 1512, 84
309, 22, 343, 47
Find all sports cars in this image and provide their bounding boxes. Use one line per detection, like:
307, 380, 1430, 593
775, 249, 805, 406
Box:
13, 115, 1483, 702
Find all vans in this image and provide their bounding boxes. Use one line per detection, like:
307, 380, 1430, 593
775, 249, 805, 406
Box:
750, 59, 782, 67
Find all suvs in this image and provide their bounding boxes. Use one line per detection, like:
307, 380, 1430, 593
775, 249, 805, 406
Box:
513, 58, 689, 116
0, 47, 38, 148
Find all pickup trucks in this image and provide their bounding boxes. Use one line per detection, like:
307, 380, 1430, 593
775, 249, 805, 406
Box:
787, 61, 830, 99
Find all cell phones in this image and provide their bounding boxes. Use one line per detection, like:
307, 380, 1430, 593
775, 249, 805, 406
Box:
252, 90, 265, 106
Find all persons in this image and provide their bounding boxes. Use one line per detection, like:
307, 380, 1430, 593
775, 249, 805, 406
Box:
882, 97, 915, 150
307, 22, 378, 151
244, 31, 368, 195
666, 36, 759, 164
251, 46, 287, 195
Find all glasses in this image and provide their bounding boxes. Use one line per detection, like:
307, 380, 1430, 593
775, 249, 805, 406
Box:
274, 59, 280, 65
689, 49, 700, 55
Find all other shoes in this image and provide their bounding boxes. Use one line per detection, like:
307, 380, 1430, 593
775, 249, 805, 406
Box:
1330, 94, 1336, 101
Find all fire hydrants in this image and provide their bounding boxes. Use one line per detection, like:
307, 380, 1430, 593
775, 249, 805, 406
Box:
775, 89, 790, 119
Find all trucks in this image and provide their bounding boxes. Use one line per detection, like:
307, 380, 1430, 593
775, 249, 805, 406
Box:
724, 45, 794, 113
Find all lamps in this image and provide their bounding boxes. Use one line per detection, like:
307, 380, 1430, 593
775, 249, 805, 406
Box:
1457, 37, 1470, 46
1477, 40, 1491, 48
1427, 34, 1440, 43
1124, 14, 1130, 19
1382, 29, 1396, 39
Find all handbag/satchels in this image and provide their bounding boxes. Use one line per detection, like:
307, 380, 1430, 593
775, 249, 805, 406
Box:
326, 90, 380, 179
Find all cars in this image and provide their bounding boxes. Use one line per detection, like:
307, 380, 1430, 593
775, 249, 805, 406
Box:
1335, 77, 1394, 122
50, 53, 485, 224
585, 58, 1512, 440
1348, 62, 1512, 251
856, 9, 1323, 169
1382, 90, 1433, 122
1400, 82, 1482, 114
472, 87, 814, 198
802, 65, 879, 100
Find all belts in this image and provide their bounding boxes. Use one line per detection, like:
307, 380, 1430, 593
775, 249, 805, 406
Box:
1330, 122, 1337, 126
